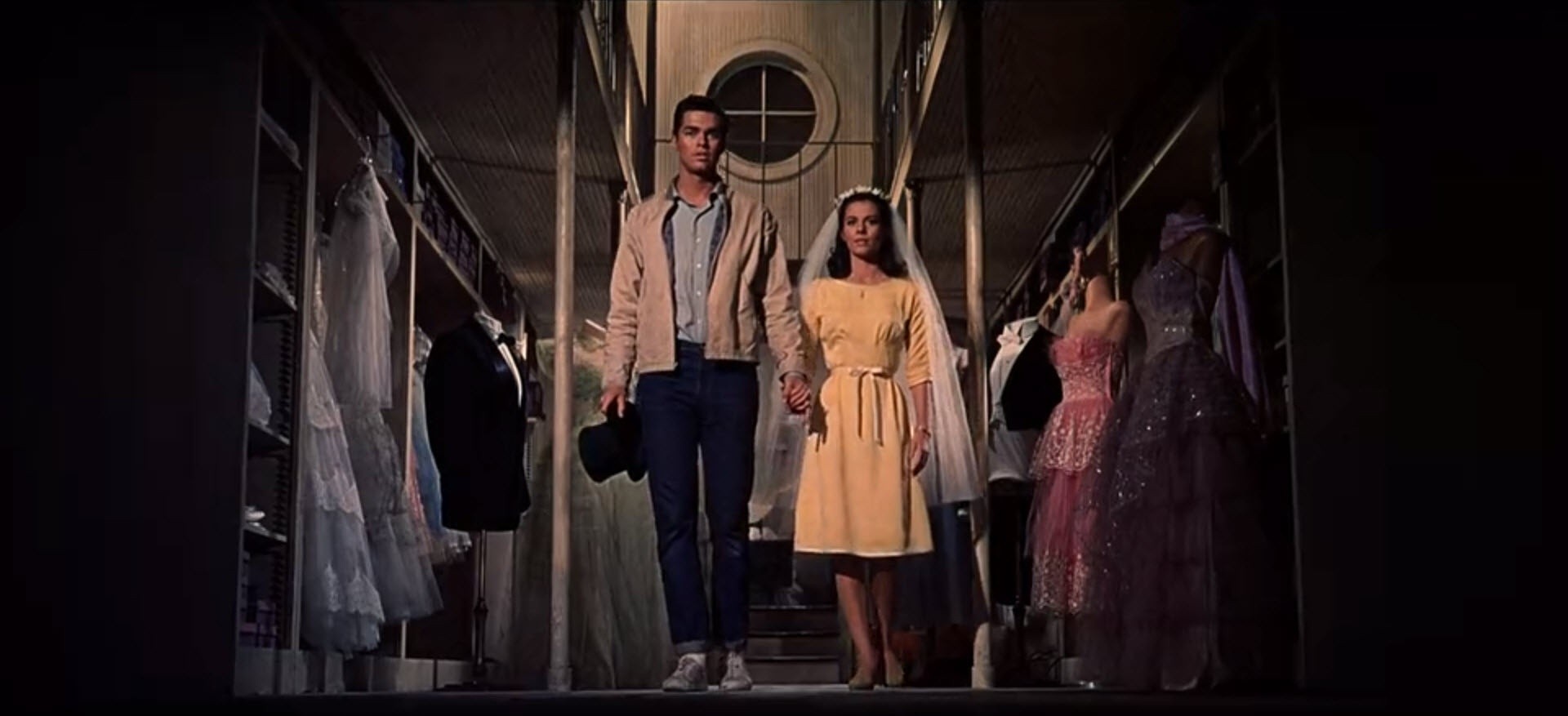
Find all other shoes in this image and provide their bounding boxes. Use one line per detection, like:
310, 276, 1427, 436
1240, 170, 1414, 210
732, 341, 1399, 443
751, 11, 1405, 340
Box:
848, 655, 885, 691
886, 659, 905, 687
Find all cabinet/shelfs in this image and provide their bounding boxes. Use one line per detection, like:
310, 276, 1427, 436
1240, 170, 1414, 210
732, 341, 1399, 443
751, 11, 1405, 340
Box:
0, 0, 527, 699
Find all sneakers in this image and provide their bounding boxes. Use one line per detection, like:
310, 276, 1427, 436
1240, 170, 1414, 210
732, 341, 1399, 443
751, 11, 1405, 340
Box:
719, 651, 753, 691
662, 657, 707, 691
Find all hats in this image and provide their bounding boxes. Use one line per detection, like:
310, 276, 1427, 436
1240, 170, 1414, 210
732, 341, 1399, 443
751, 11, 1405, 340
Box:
577, 399, 646, 483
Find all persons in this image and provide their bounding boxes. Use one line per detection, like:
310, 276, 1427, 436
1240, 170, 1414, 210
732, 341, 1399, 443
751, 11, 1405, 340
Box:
735, 183, 989, 689
1028, 273, 1137, 623
427, 308, 535, 535
511, 318, 677, 684
1076, 205, 1286, 693
600, 94, 813, 693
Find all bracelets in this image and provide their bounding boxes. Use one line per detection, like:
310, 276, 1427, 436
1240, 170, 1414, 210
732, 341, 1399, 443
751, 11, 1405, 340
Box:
916, 425, 931, 438
